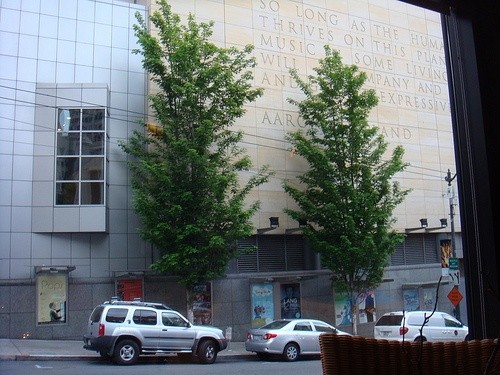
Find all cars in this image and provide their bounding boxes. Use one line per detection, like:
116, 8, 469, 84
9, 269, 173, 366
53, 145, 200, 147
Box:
245, 318, 355, 362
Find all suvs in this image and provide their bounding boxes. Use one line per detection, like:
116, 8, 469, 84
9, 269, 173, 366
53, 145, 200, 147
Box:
83, 296, 229, 366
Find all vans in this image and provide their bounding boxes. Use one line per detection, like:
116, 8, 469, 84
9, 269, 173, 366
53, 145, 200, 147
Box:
373, 310, 474, 343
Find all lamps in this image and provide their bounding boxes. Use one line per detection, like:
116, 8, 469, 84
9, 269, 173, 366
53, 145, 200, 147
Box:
425, 219, 447, 232
257, 216, 279, 235
405, 218, 428, 234
285, 220, 307, 234
